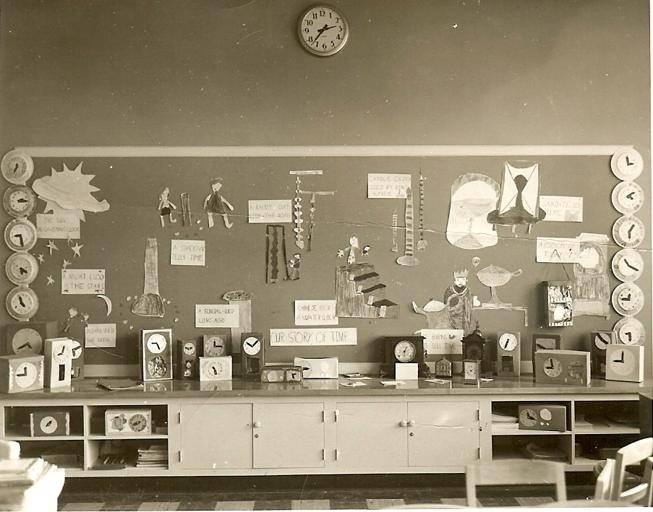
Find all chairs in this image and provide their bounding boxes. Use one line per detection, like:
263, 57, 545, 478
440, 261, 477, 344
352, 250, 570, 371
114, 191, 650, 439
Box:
466, 437, 652, 508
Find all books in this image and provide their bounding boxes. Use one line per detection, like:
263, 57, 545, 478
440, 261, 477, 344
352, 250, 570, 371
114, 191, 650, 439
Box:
135, 440, 167, 470
492, 412, 520, 424
606, 411, 638, 424
1, 456, 68, 511
528, 449, 568, 459
492, 422, 520, 430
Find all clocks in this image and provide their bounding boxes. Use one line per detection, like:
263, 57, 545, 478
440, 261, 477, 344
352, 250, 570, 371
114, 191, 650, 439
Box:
2, 150, 81, 396
138, 329, 339, 384
295, 3, 351, 58
